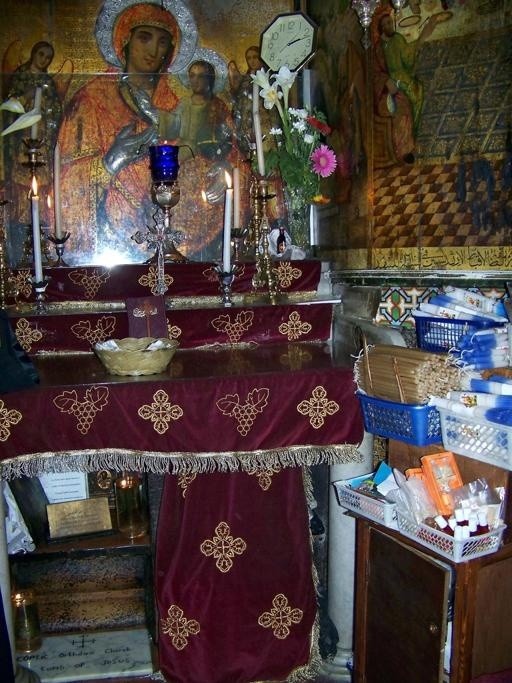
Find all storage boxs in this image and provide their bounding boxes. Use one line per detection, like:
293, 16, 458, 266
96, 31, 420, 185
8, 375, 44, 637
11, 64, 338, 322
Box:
332, 318, 512, 565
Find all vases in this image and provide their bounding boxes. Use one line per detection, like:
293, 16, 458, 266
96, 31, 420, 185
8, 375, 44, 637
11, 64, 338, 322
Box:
284, 185, 311, 254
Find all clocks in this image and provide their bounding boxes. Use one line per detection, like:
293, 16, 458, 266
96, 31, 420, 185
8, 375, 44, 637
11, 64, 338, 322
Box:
258, 12, 319, 73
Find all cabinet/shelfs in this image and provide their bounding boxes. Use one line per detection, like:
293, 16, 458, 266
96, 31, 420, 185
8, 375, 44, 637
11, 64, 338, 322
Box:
344, 437, 512, 683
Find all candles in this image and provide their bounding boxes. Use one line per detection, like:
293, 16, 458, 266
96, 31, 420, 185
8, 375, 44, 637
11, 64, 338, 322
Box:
31, 176, 47, 282
232, 158, 242, 230
53, 139, 64, 240
150, 137, 180, 184
223, 170, 233, 276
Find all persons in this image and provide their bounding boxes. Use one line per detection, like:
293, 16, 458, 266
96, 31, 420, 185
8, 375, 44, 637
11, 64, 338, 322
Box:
1, 39, 67, 160
345, 0, 456, 177
45, 1, 255, 270
233, 42, 280, 153
122, 48, 230, 163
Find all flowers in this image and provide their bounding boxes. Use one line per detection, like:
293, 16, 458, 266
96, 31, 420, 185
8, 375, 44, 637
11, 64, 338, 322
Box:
249, 66, 337, 247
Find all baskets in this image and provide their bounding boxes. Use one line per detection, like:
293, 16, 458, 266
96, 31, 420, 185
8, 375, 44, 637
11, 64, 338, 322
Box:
397, 507, 506, 558
355, 392, 442, 447
415, 311, 508, 350
441, 408, 512, 470
333, 474, 395, 528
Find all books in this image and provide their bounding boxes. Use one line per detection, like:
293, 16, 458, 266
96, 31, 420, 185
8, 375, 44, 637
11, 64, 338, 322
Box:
125, 294, 171, 340
